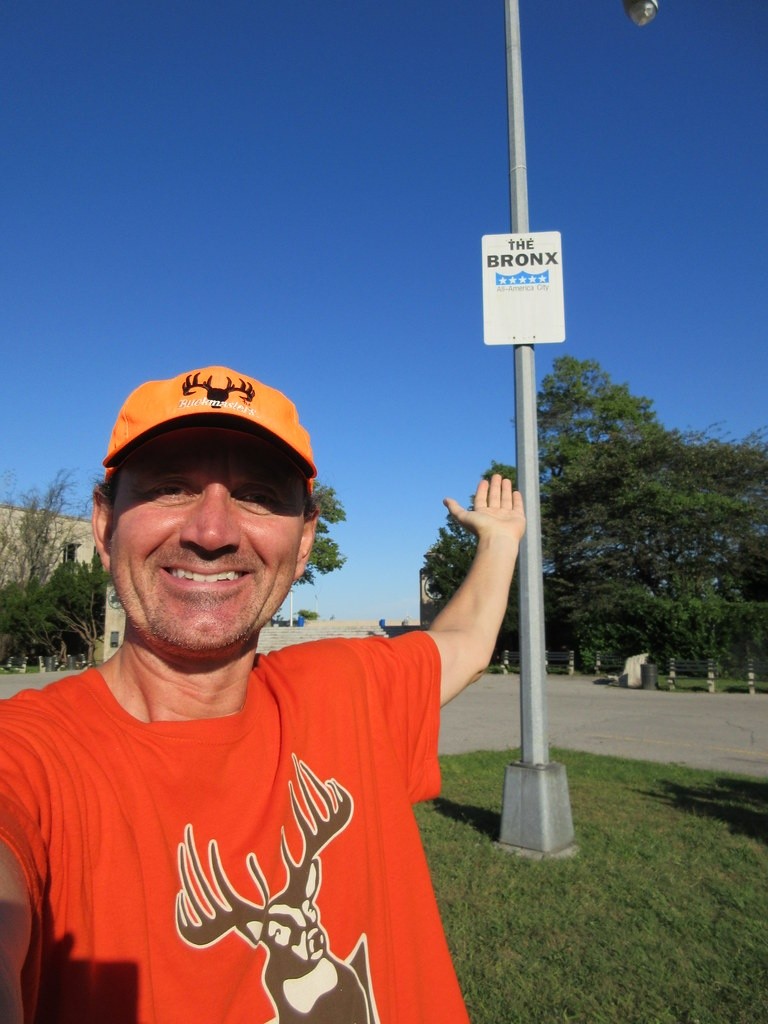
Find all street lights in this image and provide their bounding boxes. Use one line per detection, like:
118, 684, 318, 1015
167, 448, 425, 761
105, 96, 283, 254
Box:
499, 0, 661, 853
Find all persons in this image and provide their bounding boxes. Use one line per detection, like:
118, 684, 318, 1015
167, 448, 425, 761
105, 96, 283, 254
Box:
0, 366, 525, 1024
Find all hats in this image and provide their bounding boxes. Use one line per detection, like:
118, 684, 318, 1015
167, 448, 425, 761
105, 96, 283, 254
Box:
103, 367, 318, 497
